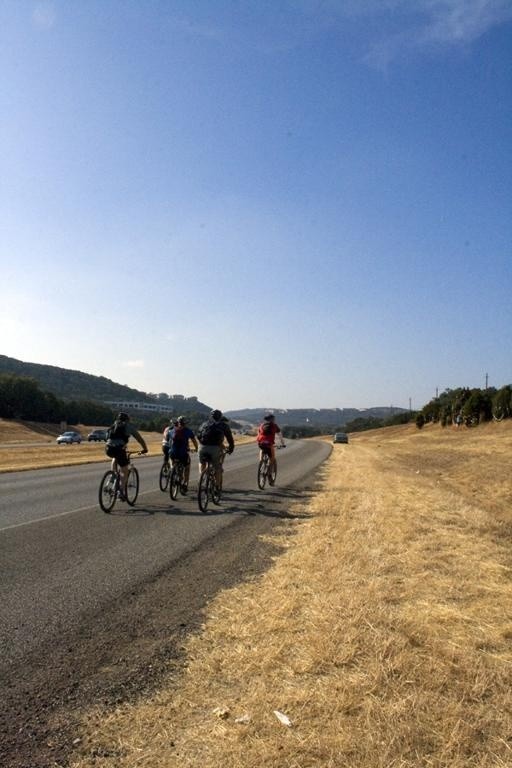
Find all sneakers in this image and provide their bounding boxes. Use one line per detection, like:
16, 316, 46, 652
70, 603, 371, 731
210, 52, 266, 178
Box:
180, 484, 190, 496
104, 485, 114, 492
266, 464, 272, 475
211, 472, 217, 484
119, 493, 127, 502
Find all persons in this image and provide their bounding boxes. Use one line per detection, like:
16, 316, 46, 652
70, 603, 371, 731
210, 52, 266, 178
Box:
161, 417, 177, 464
256, 414, 286, 475
104, 411, 148, 503
167, 416, 200, 496
197, 409, 234, 496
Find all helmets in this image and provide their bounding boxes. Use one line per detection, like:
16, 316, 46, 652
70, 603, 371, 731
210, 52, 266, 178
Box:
210, 409, 229, 422
170, 416, 191, 425
265, 414, 275, 421
119, 412, 129, 420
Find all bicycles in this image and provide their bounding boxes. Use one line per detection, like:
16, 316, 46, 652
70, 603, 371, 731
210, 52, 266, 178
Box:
97, 443, 287, 511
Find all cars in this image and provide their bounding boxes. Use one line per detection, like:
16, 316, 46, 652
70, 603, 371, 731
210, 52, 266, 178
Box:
333, 431, 349, 444
56, 431, 82, 445
88, 429, 108, 443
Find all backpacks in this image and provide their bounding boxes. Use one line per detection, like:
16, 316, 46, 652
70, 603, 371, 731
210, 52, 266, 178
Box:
202, 423, 222, 446
174, 427, 186, 442
107, 419, 130, 440
263, 423, 273, 435
